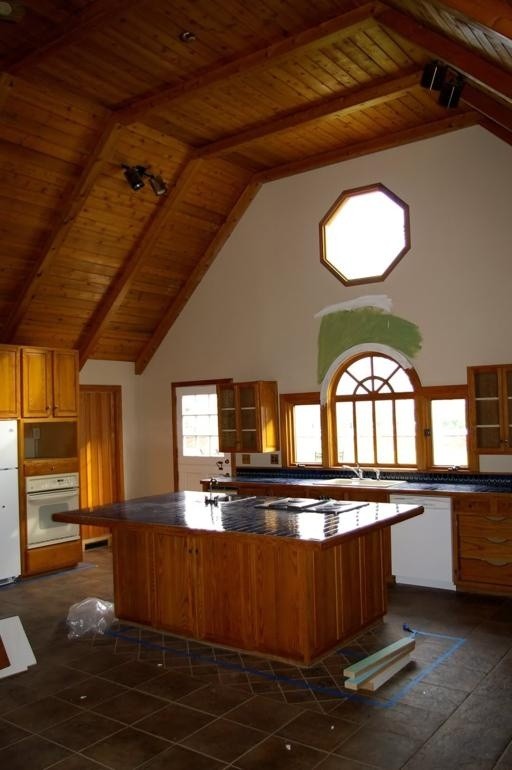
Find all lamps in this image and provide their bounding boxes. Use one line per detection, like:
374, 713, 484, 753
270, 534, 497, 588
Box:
120, 164, 167, 196
420, 59, 467, 108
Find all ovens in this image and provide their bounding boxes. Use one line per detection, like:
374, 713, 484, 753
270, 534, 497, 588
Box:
25, 472, 80, 551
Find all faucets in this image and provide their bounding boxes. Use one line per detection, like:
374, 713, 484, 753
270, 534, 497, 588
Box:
342, 464, 363, 479
373, 469, 380, 480
210, 503, 218, 522
208, 479, 218, 500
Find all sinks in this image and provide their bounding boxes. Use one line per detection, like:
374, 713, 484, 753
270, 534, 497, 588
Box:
312, 478, 354, 485
357, 478, 408, 487
217, 493, 256, 504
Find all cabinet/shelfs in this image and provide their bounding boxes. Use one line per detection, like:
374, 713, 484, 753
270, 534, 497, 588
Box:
237, 487, 389, 504
19, 345, 80, 418
0, 344, 19, 418
451, 496, 512, 596
216, 380, 279, 453
467, 364, 512, 455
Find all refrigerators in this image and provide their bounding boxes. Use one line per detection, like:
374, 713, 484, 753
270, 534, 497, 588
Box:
0, 419, 22, 589
